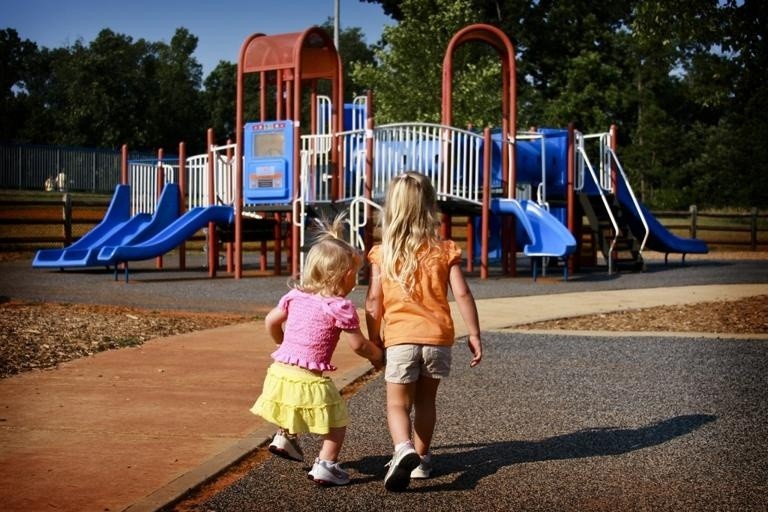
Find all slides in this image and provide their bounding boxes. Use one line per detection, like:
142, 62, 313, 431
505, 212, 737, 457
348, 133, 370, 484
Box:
617, 174, 710, 253
491, 198, 577, 257
33, 185, 233, 268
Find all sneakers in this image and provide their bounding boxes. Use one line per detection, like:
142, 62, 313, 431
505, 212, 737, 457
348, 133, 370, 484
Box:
307, 457, 350, 485
410, 455, 433, 478
268, 428, 303, 461
384, 442, 420, 492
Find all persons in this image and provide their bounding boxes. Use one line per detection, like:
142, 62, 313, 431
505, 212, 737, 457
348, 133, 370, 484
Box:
248, 210, 385, 486
45, 175, 55, 192
365, 171, 483, 491
55, 167, 70, 191
